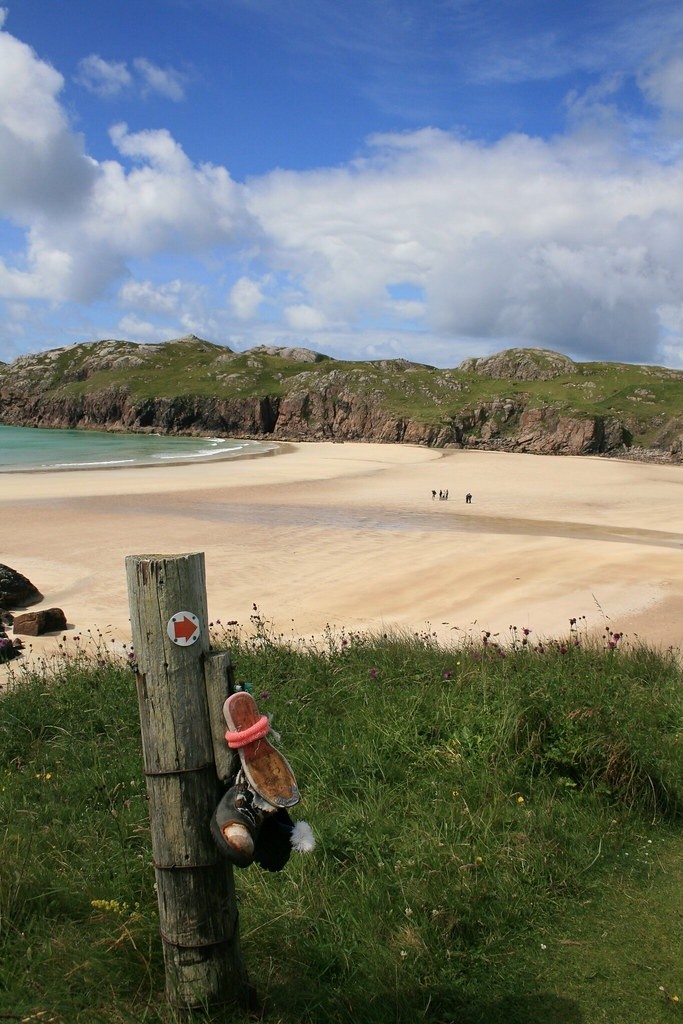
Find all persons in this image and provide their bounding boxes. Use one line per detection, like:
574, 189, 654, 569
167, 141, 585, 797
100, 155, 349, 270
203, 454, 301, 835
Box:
432, 488, 448, 501
465, 493, 472, 503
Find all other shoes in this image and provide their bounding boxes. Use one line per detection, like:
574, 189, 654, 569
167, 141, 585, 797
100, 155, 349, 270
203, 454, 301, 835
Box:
209, 782, 257, 864
252, 795, 315, 872
